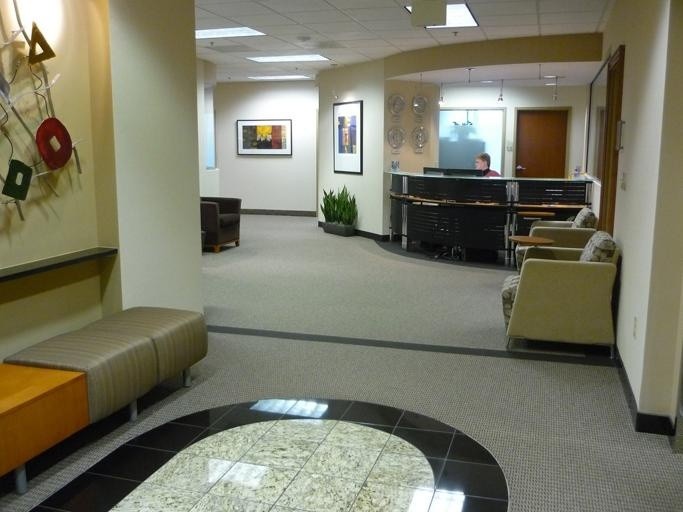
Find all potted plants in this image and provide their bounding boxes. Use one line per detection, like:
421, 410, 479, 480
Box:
237, 119, 292, 155
333, 100, 363, 176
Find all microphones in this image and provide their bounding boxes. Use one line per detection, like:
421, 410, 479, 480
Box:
85, 306, 207, 387
4, 328, 159, 422
0, 361, 90, 495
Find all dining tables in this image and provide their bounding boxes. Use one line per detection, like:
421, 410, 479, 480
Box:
320, 185, 358, 237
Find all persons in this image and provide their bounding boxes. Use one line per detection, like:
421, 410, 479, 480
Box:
475, 153, 498, 176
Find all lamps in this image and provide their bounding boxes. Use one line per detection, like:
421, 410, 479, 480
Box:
200, 197, 242, 253
528, 208, 594, 248
501, 232, 620, 359
